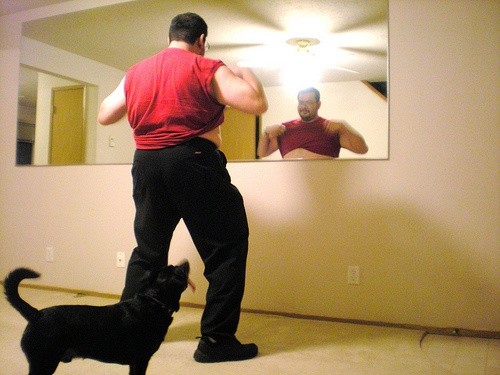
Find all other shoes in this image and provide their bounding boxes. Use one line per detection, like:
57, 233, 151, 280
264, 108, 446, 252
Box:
194, 333, 258, 363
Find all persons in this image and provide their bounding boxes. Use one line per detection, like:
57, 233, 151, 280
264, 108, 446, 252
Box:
257, 88, 368, 158
98, 12, 268, 363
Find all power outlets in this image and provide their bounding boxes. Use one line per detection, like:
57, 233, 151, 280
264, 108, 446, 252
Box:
347, 266, 359, 284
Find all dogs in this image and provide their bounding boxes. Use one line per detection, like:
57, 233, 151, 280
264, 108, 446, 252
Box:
2, 259, 196, 375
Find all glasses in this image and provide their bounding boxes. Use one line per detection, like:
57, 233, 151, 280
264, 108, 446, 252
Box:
199, 33, 211, 52
298, 100, 318, 105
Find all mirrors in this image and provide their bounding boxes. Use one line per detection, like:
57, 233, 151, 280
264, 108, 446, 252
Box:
15, 0, 390, 168
